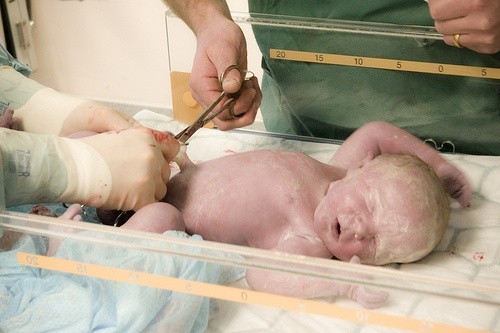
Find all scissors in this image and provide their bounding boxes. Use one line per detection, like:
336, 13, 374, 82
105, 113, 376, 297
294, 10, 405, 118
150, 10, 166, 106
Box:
170, 63, 261, 143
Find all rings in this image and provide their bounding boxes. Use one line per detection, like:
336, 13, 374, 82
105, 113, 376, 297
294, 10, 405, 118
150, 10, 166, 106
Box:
453, 34, 462, 48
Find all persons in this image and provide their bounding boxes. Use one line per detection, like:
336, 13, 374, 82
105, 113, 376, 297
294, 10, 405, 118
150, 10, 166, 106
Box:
40, 120, 473, 307
161, 0, 498, 157
0, 42, 181, 212
